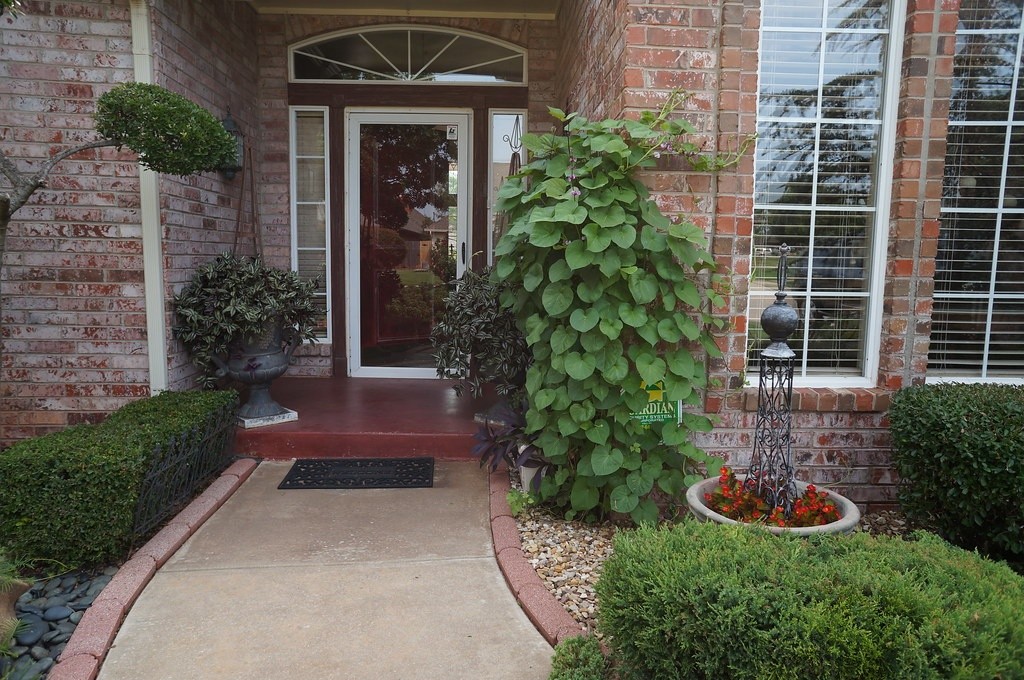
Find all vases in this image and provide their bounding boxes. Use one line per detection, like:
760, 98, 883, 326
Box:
686, 473, 861, 546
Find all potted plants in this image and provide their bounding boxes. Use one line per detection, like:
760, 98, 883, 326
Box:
424, 249, 536, 423
167, 248, 320, 421
469, 391, 581, 495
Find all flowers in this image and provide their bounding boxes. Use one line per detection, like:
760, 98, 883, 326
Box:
704, 466, 842, 528
